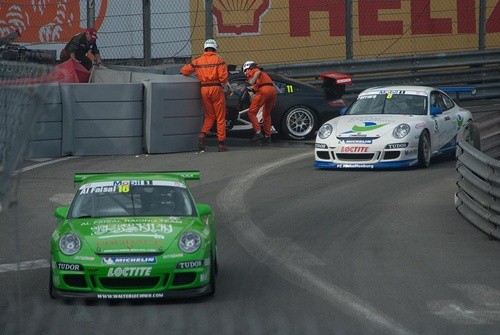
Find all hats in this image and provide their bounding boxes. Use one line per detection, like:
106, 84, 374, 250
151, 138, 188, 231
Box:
86, 28, 98, 39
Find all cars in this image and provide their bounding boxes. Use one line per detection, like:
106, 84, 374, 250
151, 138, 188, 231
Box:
48, 169, 219, 305
312, 85, 481, 172
223, 72, 347, 141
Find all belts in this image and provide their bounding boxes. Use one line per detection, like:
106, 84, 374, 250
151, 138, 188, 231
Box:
258, 83, 273, 88
201, 83, 221, 86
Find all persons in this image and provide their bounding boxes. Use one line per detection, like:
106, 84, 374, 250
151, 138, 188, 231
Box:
181, 39, 228, 152
60, 27, 106, 70
243, 59, 277, 145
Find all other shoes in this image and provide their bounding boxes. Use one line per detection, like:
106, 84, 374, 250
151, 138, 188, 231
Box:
198, 133, 206, 150
249, 131, 265, 143
218, 142, 224, 151
256, 136, 273, 146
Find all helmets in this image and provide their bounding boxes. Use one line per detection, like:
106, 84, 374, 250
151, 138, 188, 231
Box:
243, 60, 255, 74
411, 94, 435, 107
204, 39, 218, 51
155, 189, 174, 204
